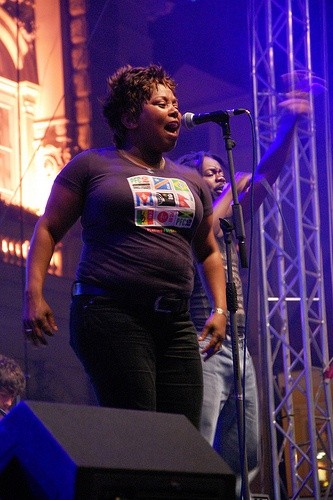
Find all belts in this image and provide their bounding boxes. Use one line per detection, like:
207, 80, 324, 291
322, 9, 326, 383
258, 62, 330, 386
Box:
74, 282, 190, 313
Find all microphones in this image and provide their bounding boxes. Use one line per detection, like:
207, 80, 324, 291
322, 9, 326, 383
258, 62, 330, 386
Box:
181, 107, 249, 128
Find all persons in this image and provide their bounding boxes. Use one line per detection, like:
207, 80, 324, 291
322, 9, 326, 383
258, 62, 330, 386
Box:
22, 63, 226, 434
175, 90, 312, 497
0, 353, 26, 420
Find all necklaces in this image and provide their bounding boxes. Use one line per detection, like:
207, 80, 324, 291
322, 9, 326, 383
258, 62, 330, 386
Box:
125, 148, 163, 170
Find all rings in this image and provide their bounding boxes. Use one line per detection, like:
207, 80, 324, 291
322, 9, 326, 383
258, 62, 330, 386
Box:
26, 328, 32, 333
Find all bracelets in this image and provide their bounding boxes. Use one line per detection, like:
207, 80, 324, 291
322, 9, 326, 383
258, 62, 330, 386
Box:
212, 308, 227, 319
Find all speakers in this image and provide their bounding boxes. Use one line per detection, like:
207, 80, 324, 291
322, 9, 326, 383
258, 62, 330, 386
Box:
0, 400, 237, 500
